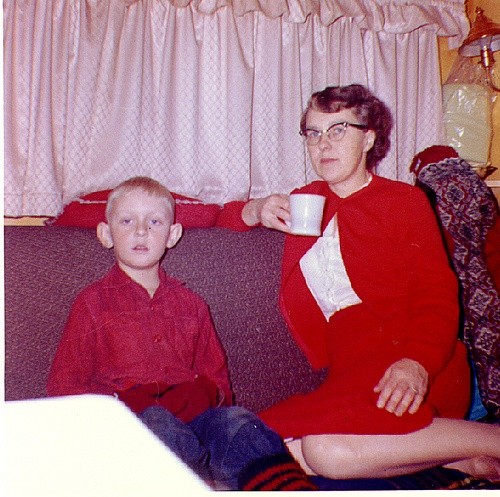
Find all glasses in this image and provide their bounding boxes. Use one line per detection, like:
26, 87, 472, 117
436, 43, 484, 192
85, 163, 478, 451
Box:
298, 122, 372, 145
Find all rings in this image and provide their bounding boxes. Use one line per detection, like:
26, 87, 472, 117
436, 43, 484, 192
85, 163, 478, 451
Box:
412, 387, 417, 393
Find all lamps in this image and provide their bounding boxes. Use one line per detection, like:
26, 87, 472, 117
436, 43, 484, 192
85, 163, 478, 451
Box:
458, 6, 500, 92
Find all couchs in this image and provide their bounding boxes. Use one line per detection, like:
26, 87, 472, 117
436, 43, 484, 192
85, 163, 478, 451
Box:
4, 225, 328, 414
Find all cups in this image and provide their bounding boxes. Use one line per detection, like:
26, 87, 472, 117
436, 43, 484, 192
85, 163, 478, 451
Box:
284, 194, 326, 236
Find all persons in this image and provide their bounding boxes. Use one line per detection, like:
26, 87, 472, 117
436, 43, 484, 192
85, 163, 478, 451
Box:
215, 84, 500, 479
45, 177, 318, 491
